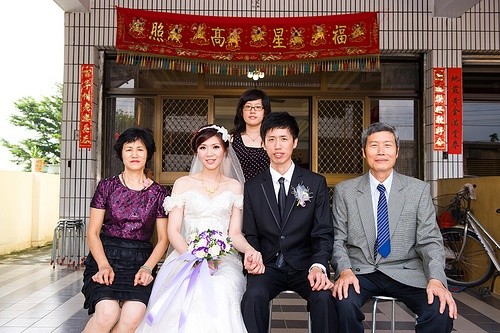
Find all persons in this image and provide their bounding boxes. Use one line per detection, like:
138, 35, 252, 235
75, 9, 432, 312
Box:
332, 123, 458, 333
231, 89, 270, 182
136, 124, 263, 333
82, 127, 168, 333
241, 112, 337, 333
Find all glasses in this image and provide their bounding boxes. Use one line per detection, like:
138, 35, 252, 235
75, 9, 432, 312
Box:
242, 104, 265, 111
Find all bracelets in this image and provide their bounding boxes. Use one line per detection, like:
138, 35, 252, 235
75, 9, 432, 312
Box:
141, 266, 152, 272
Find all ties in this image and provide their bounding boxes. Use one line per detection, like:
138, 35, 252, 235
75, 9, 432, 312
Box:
278, 177, 287, 225
376, 183, 391, 258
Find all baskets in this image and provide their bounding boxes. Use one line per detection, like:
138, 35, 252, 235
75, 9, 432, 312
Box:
432, 193, 460, 229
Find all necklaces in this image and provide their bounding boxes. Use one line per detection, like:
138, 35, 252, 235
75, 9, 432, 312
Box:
122, 172, 147, 190
202, 176, 220, 195
246, 132, 260, 142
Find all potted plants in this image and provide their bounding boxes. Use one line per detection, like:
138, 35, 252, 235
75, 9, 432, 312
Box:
46, 156, 59, 174
29, 145, 43, 172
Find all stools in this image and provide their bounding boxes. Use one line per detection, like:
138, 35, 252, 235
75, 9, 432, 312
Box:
367, 296, 402, 333
268, 290, 312, 333
50, 219, 87, 270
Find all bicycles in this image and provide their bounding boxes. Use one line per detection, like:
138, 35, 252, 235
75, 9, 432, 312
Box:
433, 183, 500, 297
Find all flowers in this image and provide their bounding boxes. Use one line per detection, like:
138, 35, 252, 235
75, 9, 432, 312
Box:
218, 127, 231, 142
184, 229, 237, 276
291, 181, 314, 208
